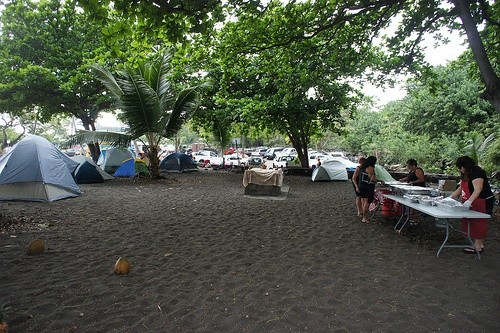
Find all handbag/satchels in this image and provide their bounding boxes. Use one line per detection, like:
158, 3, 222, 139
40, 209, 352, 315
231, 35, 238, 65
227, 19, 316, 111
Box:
361, 172, 377, 183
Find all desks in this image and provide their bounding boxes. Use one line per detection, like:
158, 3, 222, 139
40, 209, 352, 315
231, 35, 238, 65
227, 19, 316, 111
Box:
382, 194, 491, 259
372, 181, 458, 224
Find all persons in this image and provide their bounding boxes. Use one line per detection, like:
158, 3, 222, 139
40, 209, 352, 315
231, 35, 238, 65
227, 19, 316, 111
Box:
351, 155, 385, 224
449, 157, 495, 253
137, 152, 150, 168
398, 158, 426, 226
316, 159, 321, 167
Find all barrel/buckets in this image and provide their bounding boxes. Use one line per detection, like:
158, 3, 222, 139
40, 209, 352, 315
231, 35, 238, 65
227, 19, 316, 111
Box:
381, 199, 397, 216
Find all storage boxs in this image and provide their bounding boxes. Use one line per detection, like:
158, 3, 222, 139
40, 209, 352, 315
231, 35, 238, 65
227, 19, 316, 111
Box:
439, 180, 456, 191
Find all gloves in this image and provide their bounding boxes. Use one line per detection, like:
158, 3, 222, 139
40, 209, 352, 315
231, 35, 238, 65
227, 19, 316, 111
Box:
462, 200, 472, 210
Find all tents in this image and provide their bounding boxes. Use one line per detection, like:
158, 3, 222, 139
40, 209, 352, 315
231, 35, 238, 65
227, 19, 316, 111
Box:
157, 152, 200, 174
70, 156, 115, 184
0, 134, 82, 203
311, 159, 349, 181
97, 146, 156, 180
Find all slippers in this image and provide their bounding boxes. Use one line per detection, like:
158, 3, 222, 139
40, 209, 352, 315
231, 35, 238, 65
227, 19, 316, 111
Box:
463, 247, 484, 254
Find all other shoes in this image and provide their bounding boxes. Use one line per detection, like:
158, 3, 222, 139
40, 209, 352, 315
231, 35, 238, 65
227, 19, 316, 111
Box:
362, 218, 370, 223
357, 213, 363, 217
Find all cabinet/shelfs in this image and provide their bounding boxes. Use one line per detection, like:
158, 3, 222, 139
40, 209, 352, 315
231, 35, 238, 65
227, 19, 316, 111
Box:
245, 168, 282, 196
